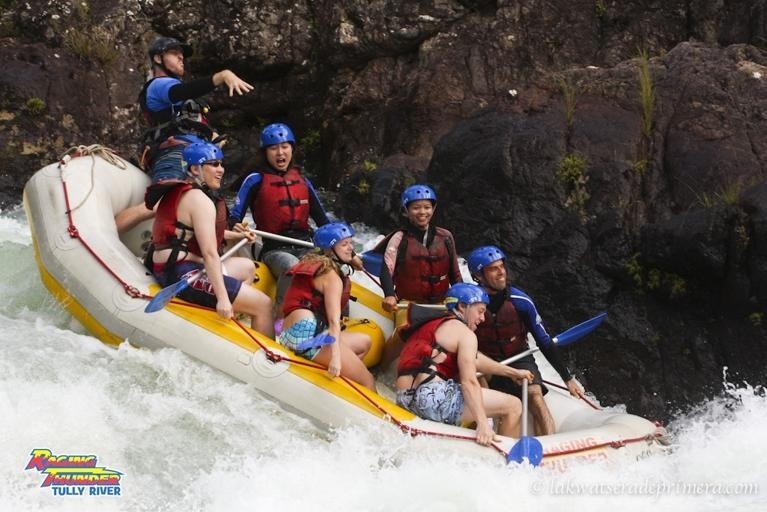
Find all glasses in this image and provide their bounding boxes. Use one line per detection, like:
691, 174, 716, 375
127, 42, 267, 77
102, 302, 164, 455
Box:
202, 162, 224, 167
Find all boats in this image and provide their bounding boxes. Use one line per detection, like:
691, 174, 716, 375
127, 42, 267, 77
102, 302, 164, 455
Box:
22, 143, 666, 464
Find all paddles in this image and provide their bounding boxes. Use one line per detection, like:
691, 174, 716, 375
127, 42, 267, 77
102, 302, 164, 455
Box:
144, 237, 248, 313
248, 228, 383, 277
507, 378, 542, 467
293, 319, 369, 353
475, 313, 606, 378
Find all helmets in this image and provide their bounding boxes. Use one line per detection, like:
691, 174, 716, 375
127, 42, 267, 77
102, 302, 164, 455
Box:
401, 185, 437, 208
183, 141, 224, 168
313, 222, 354, 250
148, 36, 194, 57
444, 283, 490, 310
468, 246, 507, 276
259, 123, 295, 149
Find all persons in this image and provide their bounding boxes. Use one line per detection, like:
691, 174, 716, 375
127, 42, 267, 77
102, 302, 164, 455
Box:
396, 282, 522, 447
279, 223, 379, 395
152, 141, 276, 341
115, 37, 254, 234
380, 185, 464, 313
229, 123, 332, 318
467, 246, 585, 436
